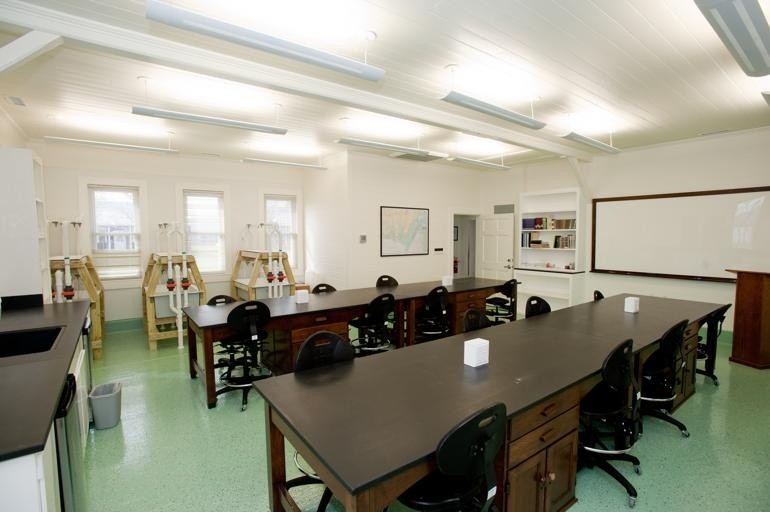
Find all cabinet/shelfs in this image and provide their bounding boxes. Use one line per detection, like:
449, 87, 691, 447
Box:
0, 148, 54, 305
0, 313, 91, 512
512, 188, 584, 307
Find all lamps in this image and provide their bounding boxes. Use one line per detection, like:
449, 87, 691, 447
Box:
694, 0, 770, 77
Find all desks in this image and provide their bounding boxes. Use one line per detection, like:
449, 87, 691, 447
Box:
183, 277, 522, 407
253, 294, 731, 512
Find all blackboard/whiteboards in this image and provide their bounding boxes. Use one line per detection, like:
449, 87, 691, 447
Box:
590, 186, 770, 283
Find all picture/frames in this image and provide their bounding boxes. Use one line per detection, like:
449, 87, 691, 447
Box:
454, 226, 458, 241
380, 206, 429, 257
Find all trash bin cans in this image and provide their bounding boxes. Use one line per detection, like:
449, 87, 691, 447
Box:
89, 382, 122, 429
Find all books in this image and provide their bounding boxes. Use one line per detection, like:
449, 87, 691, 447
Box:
521, 216, 576, 249
